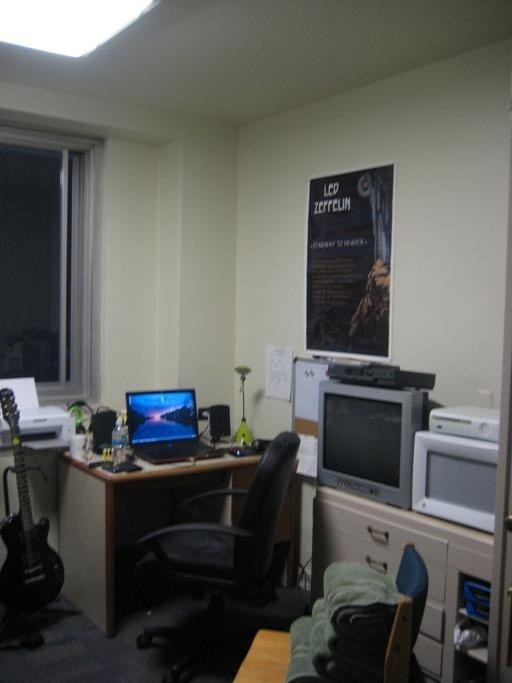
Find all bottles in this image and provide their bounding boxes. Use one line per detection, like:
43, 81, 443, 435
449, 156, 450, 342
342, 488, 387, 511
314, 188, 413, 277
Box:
113, 419, 127, 466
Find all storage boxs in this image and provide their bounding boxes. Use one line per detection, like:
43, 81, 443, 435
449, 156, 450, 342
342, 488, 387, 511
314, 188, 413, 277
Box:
463, 578, 493, 620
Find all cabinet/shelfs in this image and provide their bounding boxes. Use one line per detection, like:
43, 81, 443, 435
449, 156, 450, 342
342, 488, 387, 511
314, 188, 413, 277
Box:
317, 502, 448, 681
449, 531, 497, 683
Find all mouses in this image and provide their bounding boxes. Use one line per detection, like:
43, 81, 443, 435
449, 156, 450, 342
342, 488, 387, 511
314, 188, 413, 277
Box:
229, 445, 247, 458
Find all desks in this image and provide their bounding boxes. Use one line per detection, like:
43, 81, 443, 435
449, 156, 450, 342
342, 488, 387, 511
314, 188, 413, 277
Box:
62, 440, 298, 636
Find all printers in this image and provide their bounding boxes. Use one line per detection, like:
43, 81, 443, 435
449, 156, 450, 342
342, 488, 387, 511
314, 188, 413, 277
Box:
0, 404, 75, 452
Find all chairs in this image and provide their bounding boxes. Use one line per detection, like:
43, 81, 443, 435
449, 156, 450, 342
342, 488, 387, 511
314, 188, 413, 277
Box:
131, 433, 304, 681
232, 544, 440, 680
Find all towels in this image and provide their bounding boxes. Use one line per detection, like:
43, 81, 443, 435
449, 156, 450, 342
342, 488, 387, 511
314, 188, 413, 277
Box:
284, 555, 409, 681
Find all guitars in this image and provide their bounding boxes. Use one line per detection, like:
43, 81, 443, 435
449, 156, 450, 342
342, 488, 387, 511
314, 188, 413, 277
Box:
1, 389, 63, 615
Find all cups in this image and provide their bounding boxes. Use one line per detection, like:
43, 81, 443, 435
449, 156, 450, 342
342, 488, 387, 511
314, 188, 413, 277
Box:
70, 434, 89, 460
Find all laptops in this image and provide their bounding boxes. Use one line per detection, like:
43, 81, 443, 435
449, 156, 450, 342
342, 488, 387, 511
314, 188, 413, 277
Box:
125, 388, 227, 464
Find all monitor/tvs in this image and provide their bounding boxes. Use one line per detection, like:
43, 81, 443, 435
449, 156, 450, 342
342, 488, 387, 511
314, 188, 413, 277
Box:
315, 380, 430, 510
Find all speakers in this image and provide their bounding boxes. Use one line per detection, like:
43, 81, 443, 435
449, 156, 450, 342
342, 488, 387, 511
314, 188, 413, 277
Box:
93, 410, 117, 455
208, 405, 231, 438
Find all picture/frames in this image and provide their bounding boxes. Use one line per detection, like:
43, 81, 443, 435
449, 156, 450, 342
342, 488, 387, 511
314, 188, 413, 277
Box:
303, 158, 397, 366
